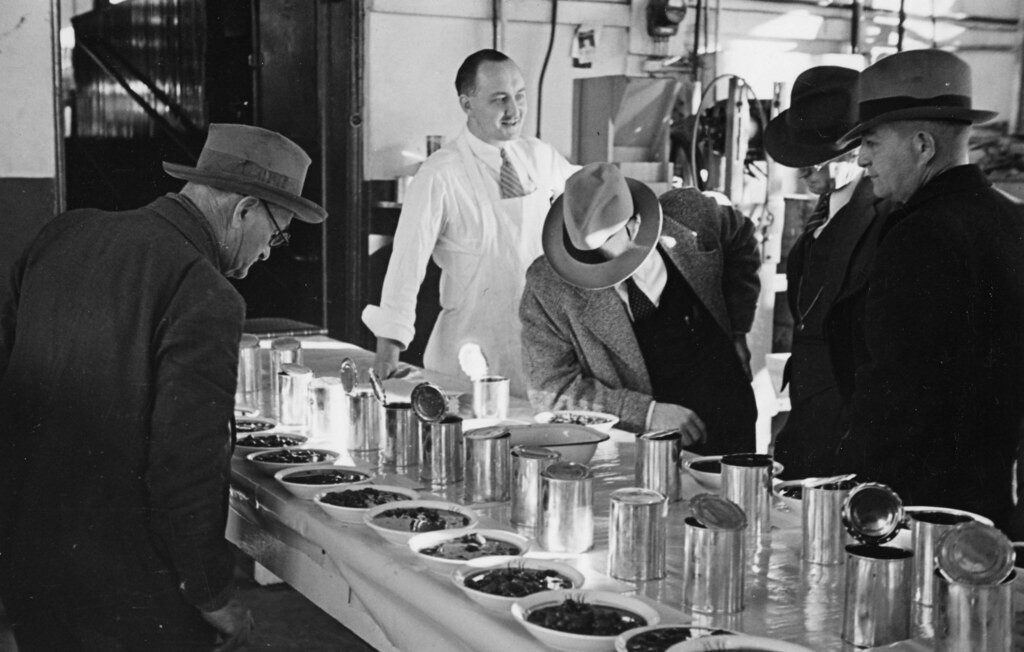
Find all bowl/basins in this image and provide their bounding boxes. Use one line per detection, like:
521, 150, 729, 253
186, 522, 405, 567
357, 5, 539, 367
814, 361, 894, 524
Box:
246, 447, 341, 478
312, 482, 419, 523
408, 528, 533, 577
363, 499, 480, 548
231, 433, 309, 457
771, 475, 865, 515
1007, 539, 1024, 590
682, 452, 784, 493
881, 504, 996, 554
231, 418, 279, 440
233, 407, 260, 420
615, 621, 744, 652
511, 585, 662, 652
465, 422, 610, 468
451, 556, 585, 613
661, 631, 814, 651
273, 466, 375, 500
534, 409, 619, 438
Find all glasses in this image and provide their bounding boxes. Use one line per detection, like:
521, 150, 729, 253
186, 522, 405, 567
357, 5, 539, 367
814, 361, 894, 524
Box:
263, 201, 289, 247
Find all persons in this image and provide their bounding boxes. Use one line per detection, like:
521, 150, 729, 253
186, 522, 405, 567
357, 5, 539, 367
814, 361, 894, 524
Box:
361, 49, 584, 399
520, 161, 761, 474
761, 50, 1024, 567
0, 123, 331, 652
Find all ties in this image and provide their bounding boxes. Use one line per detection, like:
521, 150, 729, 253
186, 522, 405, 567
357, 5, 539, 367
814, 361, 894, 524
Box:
625, 276, 698, 377
803, 178, 836, 252
500, 148, 526, 200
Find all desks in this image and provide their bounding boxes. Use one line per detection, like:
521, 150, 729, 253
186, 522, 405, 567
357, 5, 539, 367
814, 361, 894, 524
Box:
229, 335, 915, 652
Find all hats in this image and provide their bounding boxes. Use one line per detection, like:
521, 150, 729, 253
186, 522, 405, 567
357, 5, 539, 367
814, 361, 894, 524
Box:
762, 66, 862, 168
831, 48, 999, 152
163, 123, 329, 224
542, 162, 663, 291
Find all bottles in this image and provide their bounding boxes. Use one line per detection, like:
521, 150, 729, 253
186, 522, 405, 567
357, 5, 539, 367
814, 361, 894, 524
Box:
225, 335, 1019, 652
